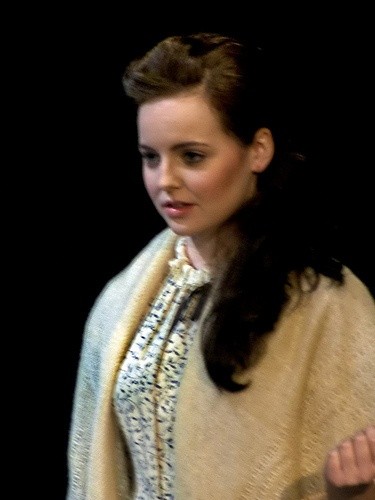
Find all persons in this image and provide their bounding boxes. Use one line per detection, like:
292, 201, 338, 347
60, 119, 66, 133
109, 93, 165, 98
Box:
65, 34, 375, 500
